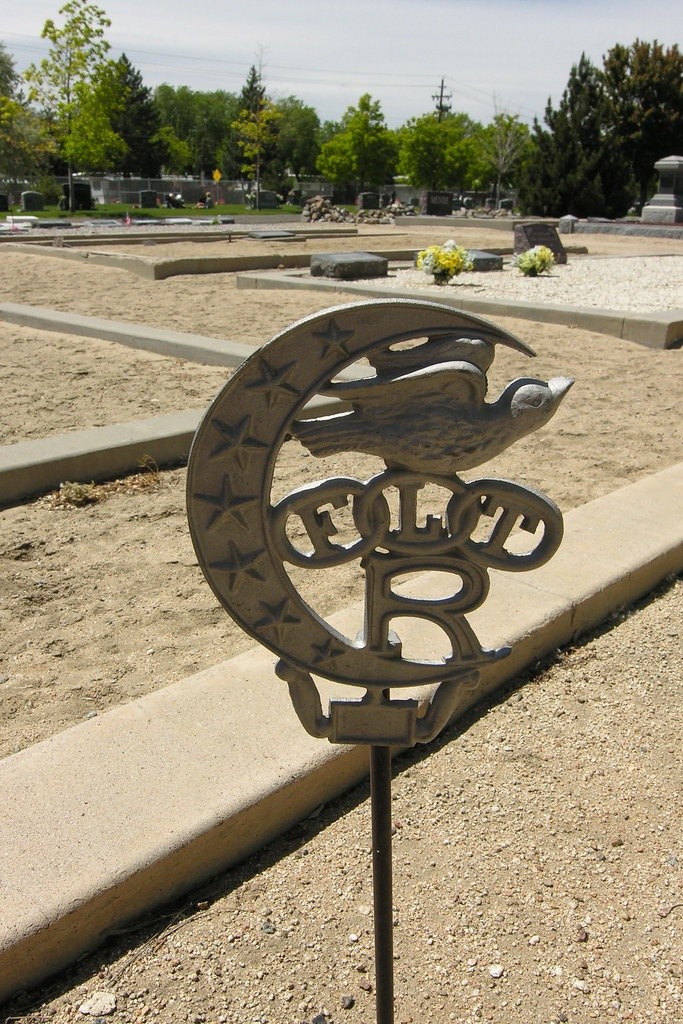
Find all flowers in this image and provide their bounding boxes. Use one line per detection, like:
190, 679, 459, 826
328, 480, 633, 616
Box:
517, 243, 556, 277
417, 238, 472, 285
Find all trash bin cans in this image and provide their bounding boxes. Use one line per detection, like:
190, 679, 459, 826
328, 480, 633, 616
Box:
300, 197, 311, 209
358, 192, 380, 209
62, 182, 92, 210
139, 190, 157, 209
